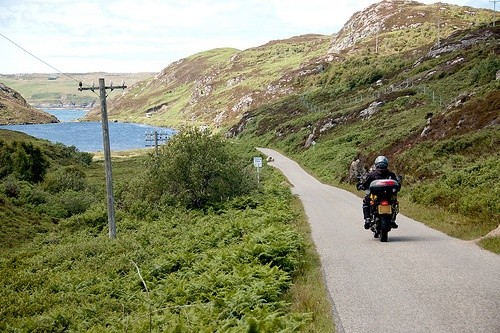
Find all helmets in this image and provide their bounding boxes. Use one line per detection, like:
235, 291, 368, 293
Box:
375, 156, 388, 169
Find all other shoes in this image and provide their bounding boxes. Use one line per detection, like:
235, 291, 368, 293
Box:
365, 219, 370, 229
391, 222, 398, 228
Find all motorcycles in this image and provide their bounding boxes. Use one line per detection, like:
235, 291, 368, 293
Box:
356, 174, 403, 243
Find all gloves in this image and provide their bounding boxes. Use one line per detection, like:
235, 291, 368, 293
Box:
358, 184, 363, 190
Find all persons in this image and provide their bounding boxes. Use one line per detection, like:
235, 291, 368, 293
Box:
356, 156, 402, 229
